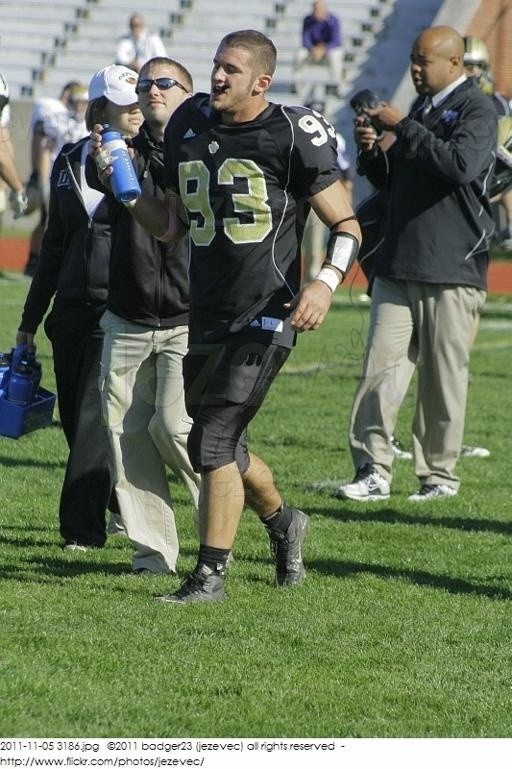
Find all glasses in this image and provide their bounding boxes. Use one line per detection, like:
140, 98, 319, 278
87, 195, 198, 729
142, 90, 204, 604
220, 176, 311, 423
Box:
135, 78, 190, 93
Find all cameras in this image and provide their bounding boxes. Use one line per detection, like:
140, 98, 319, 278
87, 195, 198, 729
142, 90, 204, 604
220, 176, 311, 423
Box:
350, 88, 387, 142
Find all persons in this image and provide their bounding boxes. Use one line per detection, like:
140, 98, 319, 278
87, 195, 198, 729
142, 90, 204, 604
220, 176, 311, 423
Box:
92, 31, 362, 606
115, 13, 168, 71
333, 25, 499, 504
84, 58, 236, 580
15, 63, 147, 549
466, 37, 512, 254
291, 2, 343, 96
0, 71, 93, 285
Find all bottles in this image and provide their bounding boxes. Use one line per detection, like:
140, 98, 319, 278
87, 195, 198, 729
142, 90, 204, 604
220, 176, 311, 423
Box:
98, 123, 142, 202
10, 361, 32, 405
31, 362, 43, 393
0, 352, 10, 395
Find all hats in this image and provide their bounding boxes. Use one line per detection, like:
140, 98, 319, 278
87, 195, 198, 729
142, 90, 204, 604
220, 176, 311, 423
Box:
89, 64, 140, 106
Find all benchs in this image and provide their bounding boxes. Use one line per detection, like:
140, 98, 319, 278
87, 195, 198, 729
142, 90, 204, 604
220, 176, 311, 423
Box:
4, 0, 393, 217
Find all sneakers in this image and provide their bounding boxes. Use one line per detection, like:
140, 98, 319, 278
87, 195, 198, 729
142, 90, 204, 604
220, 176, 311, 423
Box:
264, 508, 309, 586
154, 563, 225, 604
336, 463, 461, 501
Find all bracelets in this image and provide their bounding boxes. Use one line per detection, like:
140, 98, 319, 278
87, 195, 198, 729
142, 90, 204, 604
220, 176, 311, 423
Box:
321, 232, 359, 283
317, 267, 342, 292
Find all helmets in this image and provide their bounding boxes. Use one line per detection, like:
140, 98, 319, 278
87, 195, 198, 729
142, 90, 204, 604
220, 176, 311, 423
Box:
464, 38, 489, 67
